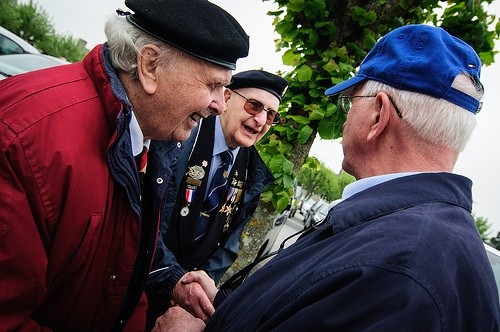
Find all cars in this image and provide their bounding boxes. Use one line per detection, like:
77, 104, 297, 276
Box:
0, 27, 72, 86
483, 243, 500, 288
258, 179, 332, 258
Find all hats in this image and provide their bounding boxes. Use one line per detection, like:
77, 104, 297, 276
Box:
324, 24, 484, 115
116, 0, 250, 71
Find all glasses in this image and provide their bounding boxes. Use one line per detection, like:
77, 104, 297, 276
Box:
233, 90, 281, 122
339, 94, 403, 119
224, 69, 289, 104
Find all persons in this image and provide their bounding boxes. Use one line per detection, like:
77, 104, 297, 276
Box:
0, 0, 250, 332
143, 71, 289, 332
151, 24, 500, 331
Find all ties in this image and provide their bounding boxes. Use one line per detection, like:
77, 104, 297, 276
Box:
193, 150, 234, 251
136, 146, 148, 195
